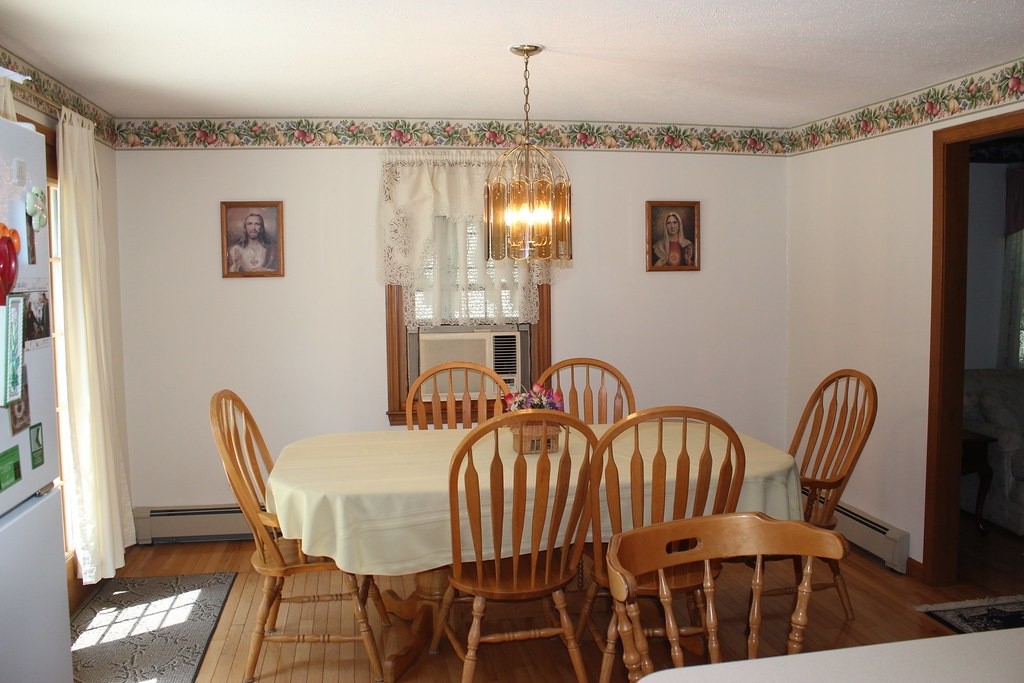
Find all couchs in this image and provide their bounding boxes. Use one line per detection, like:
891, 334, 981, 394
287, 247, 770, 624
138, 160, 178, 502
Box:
960, 368, 1024, 536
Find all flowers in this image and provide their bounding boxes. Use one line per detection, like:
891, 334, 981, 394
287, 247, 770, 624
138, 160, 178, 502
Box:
504, 383, 564, 412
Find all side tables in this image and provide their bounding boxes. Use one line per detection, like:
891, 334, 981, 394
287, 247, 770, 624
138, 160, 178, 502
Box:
957, 429, 999, 537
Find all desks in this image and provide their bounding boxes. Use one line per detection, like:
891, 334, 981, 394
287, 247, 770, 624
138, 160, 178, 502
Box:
265, 419, 806, 683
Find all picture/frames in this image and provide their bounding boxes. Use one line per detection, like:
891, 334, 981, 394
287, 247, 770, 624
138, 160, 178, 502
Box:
645, 201, 700, 272
220, 201, 284, 278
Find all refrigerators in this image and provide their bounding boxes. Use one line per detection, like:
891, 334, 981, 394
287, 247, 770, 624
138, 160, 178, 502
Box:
0, 118, 73, 683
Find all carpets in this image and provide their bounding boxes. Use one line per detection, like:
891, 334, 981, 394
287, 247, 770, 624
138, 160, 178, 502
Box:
912, 593, 1024, 634
68, 571, 239, 683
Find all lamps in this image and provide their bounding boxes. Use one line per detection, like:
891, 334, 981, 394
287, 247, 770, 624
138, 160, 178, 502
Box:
483, 45, 573, 261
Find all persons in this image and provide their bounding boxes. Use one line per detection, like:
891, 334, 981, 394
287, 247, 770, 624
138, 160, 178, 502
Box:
27, 293, 50, 339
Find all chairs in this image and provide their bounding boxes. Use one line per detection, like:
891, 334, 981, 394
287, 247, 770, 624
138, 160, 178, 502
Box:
529, 358, 635, 426
428, 409, 603, 683
209, 388, 392, 683
575, 406, 746, 683
732, 369, 878, 637
405, 361, 515, 430
605, 511, 856, 683
